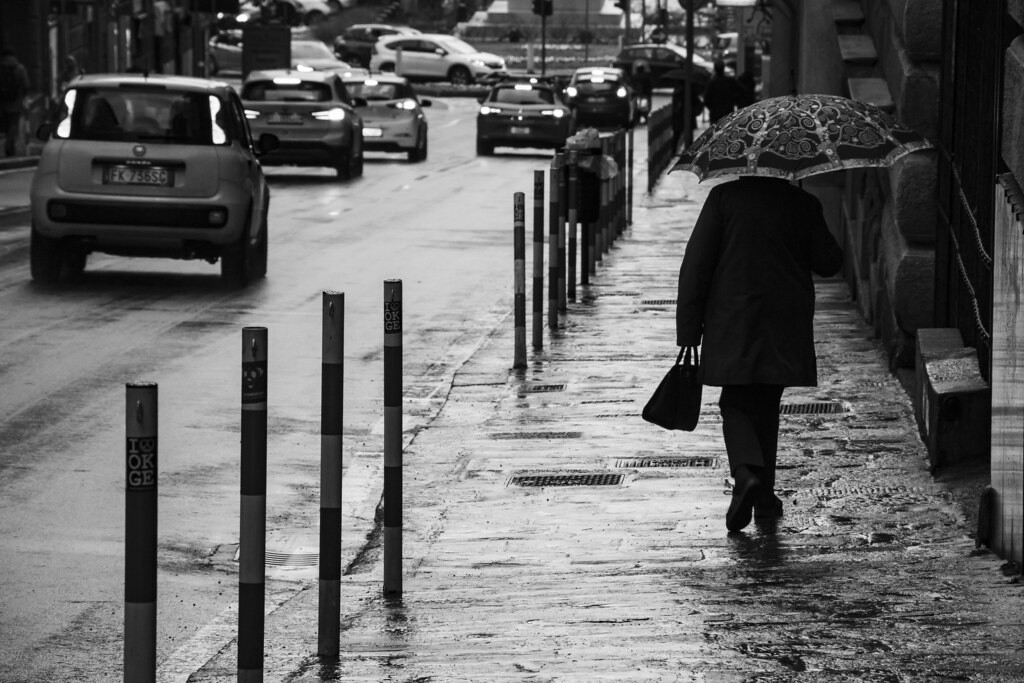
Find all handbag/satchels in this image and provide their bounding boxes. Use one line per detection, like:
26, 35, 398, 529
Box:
642, 346, 703, 432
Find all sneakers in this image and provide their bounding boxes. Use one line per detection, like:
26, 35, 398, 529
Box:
726, 476, 759, 531
754, 494, 783, 523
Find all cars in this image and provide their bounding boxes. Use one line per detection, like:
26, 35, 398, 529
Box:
566, 64, 641, 127
696, 34, 764, 74
206, 30, 242, 75
242, 69, 364, 183
287, 39, 350, 72
474, 73, 573, 156
239, 0, 353, 25
369, 34, 507, 85
332, 24, 424, 60
614, 44, 735, 85
29, 70, 271, 295
343, 73, 427, 162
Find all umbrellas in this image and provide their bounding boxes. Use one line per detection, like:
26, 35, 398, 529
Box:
667, 93, 935, 190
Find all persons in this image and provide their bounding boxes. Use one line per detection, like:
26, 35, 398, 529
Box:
736, 60, 758, 109
0, 48, 31, 159
630, 64, 654, 113
132, 0, 173, 75
677, 174, 843, 540
669, 59, 702, 158
45, 76, 69, 134
704, 60, 738, 126
